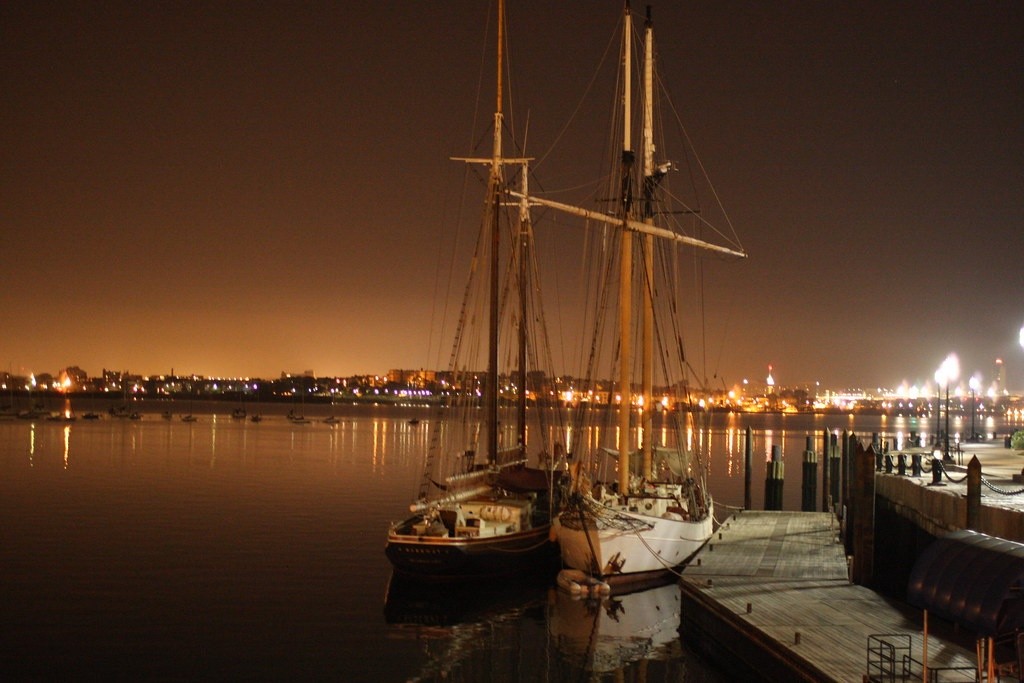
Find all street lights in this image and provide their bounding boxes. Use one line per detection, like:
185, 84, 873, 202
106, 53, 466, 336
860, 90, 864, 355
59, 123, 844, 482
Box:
931, 351, 986, 465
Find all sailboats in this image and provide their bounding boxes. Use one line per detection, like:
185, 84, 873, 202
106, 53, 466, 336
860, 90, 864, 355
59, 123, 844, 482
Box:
386, 2, 748, 597
544, 577, 684, 682
